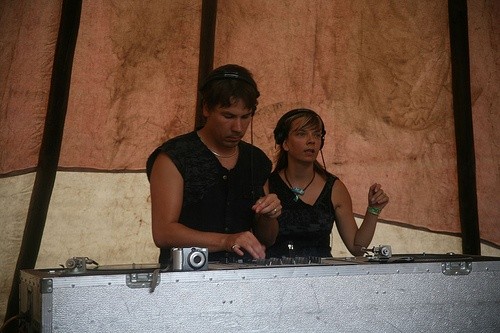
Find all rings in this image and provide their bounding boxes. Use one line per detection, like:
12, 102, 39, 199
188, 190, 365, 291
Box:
274, 208, 278, 212
231, 244, 240, 249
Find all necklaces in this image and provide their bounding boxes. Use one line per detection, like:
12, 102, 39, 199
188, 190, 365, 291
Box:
283, 166, 317, 203
196, 131, 238, 158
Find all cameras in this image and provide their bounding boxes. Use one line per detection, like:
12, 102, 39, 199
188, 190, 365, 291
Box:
172, 246, 208, 272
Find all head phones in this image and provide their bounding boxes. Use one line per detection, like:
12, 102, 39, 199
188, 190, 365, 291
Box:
200, 70, 260, 117
276, 109, 326, 151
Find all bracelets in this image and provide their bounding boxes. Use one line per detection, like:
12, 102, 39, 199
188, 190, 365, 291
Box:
366, 206, 382, 216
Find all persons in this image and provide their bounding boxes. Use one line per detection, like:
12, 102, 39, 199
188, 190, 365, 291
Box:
144, 63, 282, 262
263, 106, 389, 263
